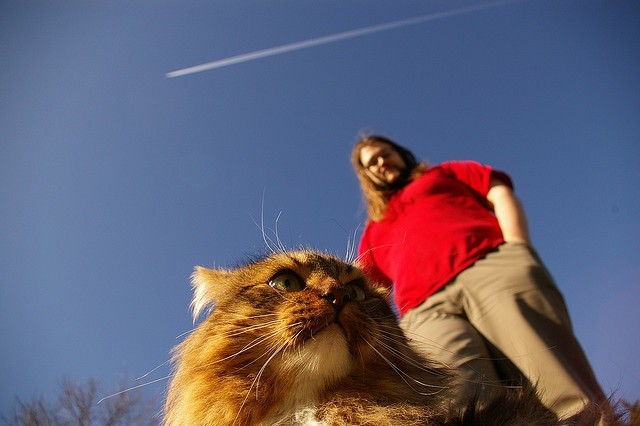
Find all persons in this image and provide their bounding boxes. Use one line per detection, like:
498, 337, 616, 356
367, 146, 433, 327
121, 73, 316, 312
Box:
348, 132, 618, 426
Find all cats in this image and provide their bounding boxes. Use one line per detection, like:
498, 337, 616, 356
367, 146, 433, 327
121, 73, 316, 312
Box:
162, 248, 640, 426
